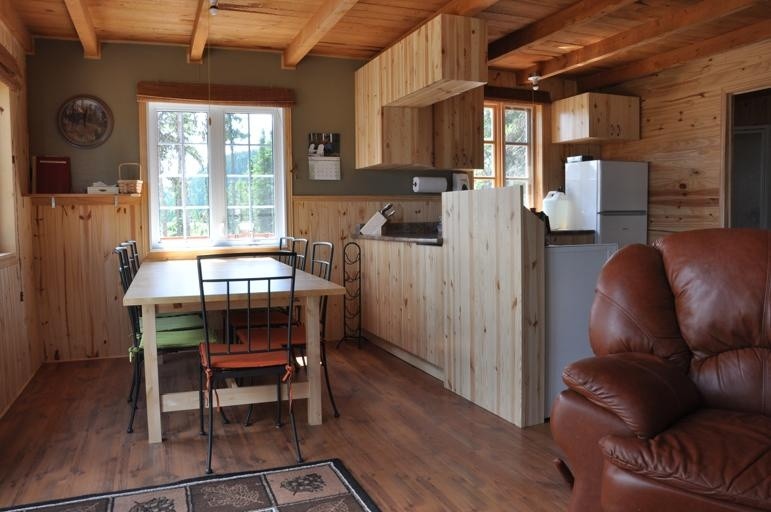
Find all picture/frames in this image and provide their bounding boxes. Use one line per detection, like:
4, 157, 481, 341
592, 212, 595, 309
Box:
57, 94, 114, 150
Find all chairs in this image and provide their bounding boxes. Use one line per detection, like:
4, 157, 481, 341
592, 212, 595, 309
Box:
545, 226, 771, 511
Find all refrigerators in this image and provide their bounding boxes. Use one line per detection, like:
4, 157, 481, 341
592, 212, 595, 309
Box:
564, 159, 649, 248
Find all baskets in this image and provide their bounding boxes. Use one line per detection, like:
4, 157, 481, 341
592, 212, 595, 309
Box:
116, 162, 144, 194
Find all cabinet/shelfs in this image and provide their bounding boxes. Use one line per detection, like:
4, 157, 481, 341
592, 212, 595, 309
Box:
354, 12, 490, 172
549, 92, 641, 146
31, 193, 147, 364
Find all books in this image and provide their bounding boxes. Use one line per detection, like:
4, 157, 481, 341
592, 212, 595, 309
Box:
309, 133, 343, 182
36, 157, 72, 193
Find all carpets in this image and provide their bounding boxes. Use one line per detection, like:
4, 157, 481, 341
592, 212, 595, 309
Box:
1, 458, 383, 512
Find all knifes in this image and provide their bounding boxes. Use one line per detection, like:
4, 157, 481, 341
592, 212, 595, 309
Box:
382, 202, 397, 218
